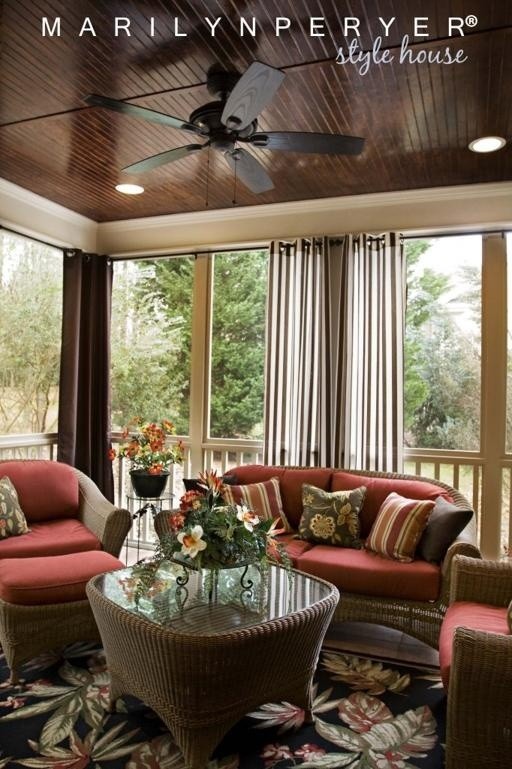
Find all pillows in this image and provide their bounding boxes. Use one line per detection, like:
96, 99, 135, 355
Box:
220, 476, 434, 562
1, 475, 34, 541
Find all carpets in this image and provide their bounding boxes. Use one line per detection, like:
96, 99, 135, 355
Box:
0, 643, 446, 769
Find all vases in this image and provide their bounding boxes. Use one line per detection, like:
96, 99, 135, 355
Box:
129, 465, 172, 497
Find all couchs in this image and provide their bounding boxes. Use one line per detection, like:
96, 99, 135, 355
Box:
154, 461, 481, 653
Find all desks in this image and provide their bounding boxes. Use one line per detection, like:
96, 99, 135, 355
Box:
124, 493, 175, 562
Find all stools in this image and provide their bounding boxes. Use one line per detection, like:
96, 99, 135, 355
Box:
0, 549, 128, 685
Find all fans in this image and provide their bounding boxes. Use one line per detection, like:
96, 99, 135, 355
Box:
75, 59, 368, 212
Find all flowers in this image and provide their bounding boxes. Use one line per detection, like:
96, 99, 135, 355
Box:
114, 562, 174, 610
104, 413, 189, 475
130, 469, 299, 605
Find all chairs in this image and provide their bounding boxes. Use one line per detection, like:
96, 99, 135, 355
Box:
438, 553, 512, 768
1, 459, 134, 557
